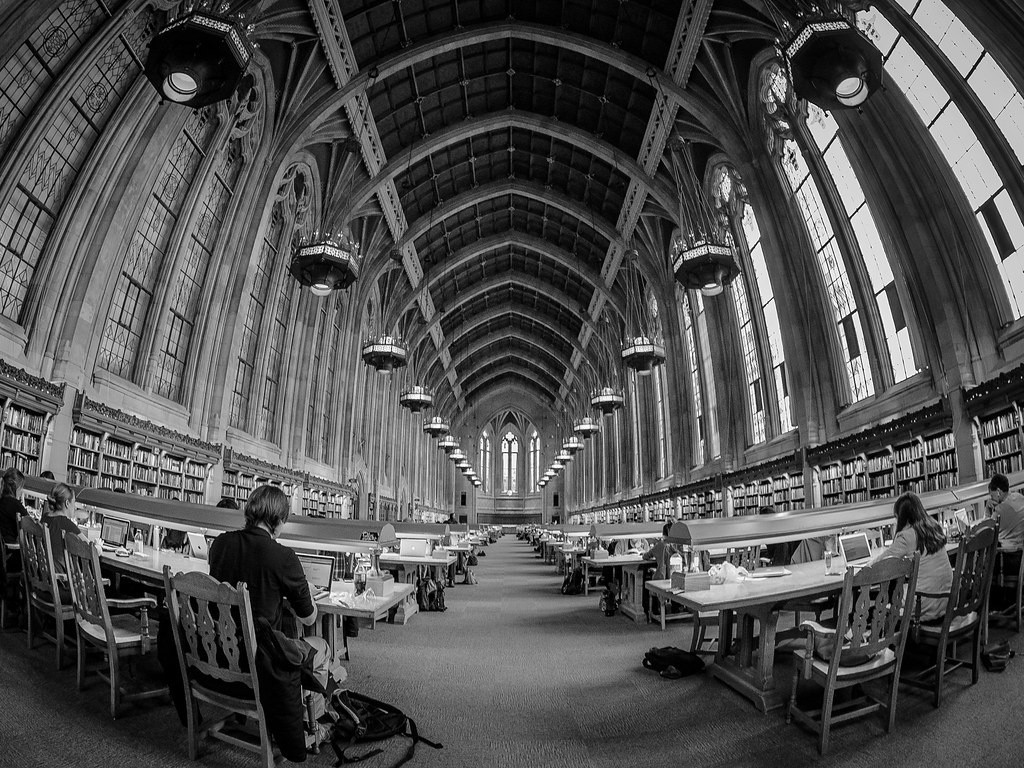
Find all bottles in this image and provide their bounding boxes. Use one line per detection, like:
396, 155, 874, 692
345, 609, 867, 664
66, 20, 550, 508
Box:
134, 529, 144, 553
354, 559, 367, 595
669, 550, 682, 585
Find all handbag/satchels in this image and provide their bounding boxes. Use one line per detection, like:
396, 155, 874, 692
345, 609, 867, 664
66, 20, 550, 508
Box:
980, 639, 1016, 673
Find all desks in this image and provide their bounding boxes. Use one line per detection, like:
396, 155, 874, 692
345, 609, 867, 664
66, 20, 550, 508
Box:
646, 541, 961, 713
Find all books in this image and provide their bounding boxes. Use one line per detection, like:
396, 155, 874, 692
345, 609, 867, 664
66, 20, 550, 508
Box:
0, 400, 44, 477
222, 471, 342, 520
983, 407, 1024, 481
572, 433, 958, 523
67, 429, 208, 505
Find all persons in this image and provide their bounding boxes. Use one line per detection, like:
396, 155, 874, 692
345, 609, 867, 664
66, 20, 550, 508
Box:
864, 491, 954, 675
39, 484, 121, 664
615, 539, 650, 556
759, 508, 802, 568
642, 522, 685, 615
436, 513, 458, 524
0, 465, 32, 573
984, 473, 1024, 576
166, 497, 187, 542
40, 471, 55, 481
208, 485, 338, 728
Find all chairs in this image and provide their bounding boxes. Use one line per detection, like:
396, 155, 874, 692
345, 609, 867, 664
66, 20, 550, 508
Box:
163, 564, 323, 768
20, 517, 103, 671
1, 513, 27, 636
62, 531, 169, 716
993, 542, 1024, 632
786, 549, 920, 753
898, 515, 1001, 708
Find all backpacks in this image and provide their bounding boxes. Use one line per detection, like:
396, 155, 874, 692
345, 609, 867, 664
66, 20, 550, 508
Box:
330, 687, 445, 768
561, 566, 582, 595
602, 590, 618, 617
467, 556, 479, 566
642, 646, 706, 680
465, 568, 478, 585
416, 577, 448, 612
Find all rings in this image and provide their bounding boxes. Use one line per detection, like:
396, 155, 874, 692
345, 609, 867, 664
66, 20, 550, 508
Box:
991, 504, 993, 505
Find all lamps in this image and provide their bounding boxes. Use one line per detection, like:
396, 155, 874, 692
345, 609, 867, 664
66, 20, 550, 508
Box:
944, 467, 1023, 504
17, 475, 399, 554
144, 1, 883, 492
667, 489, 956, 554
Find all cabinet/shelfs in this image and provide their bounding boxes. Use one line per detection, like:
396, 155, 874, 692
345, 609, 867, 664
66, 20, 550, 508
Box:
817, 428, 959, 507
367, 493, 451, 525
565, 468, 805, 525
224, 467, 305, 516
304, 486, 353, 520
0, 394, 61, 473
69, 426, 221, 503
971, 395, 1024, 479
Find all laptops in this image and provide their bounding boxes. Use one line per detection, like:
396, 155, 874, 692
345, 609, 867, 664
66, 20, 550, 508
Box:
839, 532, 874, 568
187, 532, 208, 561
294, 552, 336, 601
954, 508, 970, 534
400, 539, 426, 557
100, 515, 131, 552
